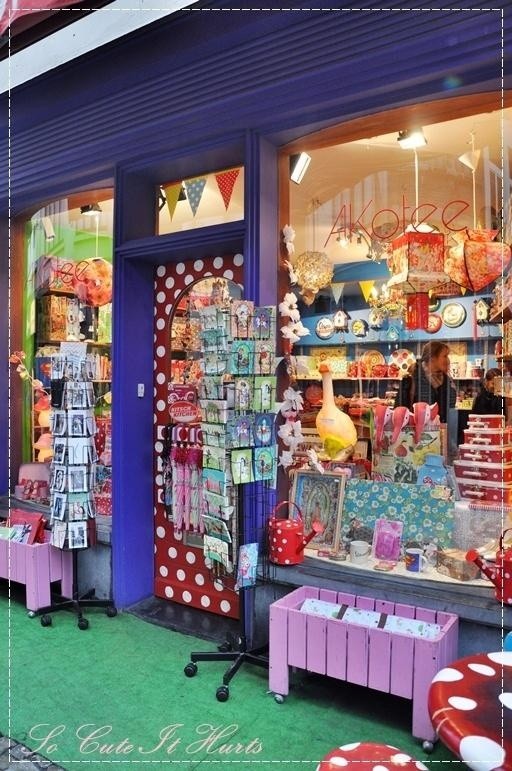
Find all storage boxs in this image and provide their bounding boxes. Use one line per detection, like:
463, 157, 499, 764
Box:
453, 501, 512, 556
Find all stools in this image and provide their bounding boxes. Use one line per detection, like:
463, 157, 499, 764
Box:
308, 743, 429, 770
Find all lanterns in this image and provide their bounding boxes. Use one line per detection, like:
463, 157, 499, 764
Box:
445, 227, 510, 293
390, 223, 446, 332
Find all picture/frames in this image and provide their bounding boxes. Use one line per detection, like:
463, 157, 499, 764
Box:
288, 466, 349, 554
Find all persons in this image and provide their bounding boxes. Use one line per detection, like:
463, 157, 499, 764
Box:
396, 341, 456, 423
472, 368, 502, 414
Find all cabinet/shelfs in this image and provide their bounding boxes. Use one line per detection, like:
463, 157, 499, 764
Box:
31, 338, 113, 461
0, 518, 80, 616
489, 254, 511, 398
291, 335, 503, 411
267, 582, 461, 751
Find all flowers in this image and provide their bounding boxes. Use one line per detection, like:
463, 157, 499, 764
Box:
8, 349, 54, 400
274, 220, 308, 472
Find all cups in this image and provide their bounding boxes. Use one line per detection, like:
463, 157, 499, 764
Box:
405, 547, 427, 575
350, 541, 374, 565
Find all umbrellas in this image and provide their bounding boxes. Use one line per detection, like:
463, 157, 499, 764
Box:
161, 425, 207, 539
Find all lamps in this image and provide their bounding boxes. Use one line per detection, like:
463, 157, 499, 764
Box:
294, 203, 333, 292
79, 202, 103, 217
292, 152, 311, 186
395, 124, 428, 150
158, 184, 187, 211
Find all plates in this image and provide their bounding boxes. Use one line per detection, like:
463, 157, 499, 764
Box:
389, 349, 416, 379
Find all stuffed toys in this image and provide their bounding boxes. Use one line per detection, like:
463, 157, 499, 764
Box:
311, 362, 443, 463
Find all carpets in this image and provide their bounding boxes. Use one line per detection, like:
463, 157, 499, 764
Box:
0, 589, 473, 770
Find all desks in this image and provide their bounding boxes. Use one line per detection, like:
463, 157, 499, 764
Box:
428, 647, 512, 770
